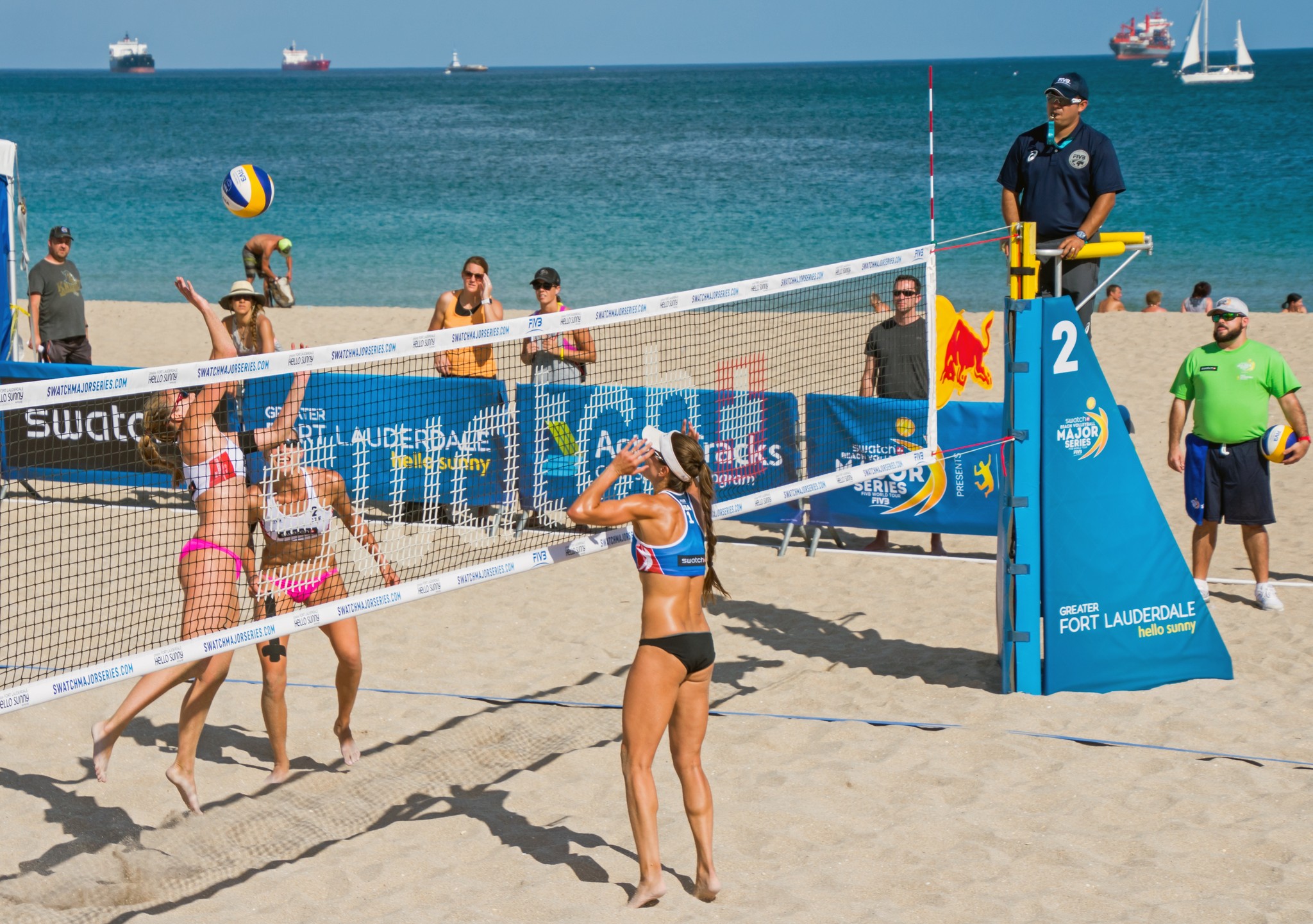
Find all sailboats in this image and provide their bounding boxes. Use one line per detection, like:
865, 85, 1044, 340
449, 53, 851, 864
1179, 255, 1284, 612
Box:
1173, 0, 1255, 85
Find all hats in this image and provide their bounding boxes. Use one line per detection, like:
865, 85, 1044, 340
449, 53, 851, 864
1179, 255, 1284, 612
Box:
529, 267, 560, 285
1207, 296, 1248, 317
641, 425, 692, 483
50, 226, 73, 241
278, 238, 292, 257
219, 280, 266, 311
1045, 72, 1089, 100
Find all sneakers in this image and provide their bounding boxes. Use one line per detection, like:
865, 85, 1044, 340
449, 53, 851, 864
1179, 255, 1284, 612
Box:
1195, 583, 1210, 603
1255, 584, 1285, 611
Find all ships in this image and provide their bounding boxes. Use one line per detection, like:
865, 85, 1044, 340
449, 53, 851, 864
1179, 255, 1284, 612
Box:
1107, 7, 1176, 62
108, 31, 156, 75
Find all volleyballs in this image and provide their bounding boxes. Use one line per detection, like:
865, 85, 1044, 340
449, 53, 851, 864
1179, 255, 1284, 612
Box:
1260, 424, 1297, 463
221, 164, 274, 218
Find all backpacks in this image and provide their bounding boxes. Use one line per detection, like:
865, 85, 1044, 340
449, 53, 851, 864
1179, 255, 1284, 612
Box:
269, 276, 295, 308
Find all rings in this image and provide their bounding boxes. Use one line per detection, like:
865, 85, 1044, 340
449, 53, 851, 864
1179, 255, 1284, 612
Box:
1071, 248, 1075, 250
630, 449, 634, 453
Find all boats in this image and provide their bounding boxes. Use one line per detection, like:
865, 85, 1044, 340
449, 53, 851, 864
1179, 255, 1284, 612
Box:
281, 39, 331, 71
1149, 59, 1169, 67
444, 49, 490, 72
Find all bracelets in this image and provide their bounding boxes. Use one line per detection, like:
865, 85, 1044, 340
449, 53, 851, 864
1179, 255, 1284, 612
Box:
86, 324, 88, 327
275, 277, 278, 281
561, 347, 563, 360
248, 575, 258, 583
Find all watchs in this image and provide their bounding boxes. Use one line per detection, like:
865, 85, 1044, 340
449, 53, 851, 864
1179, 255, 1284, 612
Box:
1074, 230, 1088, 244
1298, 435, 1312, 443
481, 298, 492, 305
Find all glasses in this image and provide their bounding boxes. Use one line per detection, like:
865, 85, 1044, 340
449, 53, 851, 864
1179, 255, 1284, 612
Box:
533, 282, 552, 290
1046, 93, 1081, 106
894, 290, 918, 297
272, 439, 300, 449
1212, 313, 1245, 322
165, 389, 188, 427
463, 270, 484, 281
233, 296, 251, 301
645, 447, 662, 460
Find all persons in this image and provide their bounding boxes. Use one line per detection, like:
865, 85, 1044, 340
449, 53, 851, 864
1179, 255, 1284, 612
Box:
240, 428, 400, 784
566, 418, 730, 908
242, 234, 293, 307
207, 280, 279, 360
996, 71, 1126, 343
859, 274, 929, 400
1167, 297, 1311, 612
427, 256, 503, 379
1141, 290, 1168, 312
26, 226, 92, 365
1181, 281, 1213, 313
1097, 284, 1126, 313
520, 267, 598, 385
1281, 293, 1307, 313
871, 291, 890, 312
90, 276, 311, 815
